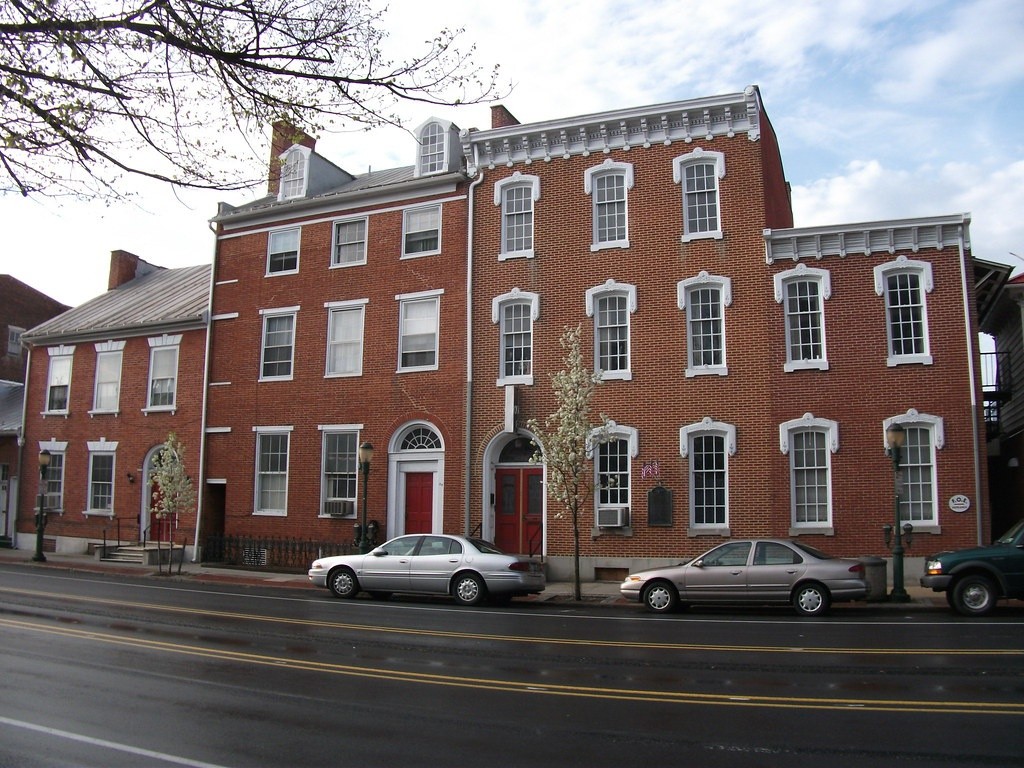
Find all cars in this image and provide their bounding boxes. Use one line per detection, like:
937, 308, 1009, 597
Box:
620, 538, 872, 618
308, 534, 545, 607
919, 519, 1024, 619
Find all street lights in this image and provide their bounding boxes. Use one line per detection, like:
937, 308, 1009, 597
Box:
31, 450, 52, 561
359, 440, 373, 555
885, 423, 912, 603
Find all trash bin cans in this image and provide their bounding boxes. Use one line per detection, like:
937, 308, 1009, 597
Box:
859, 557, 887, 602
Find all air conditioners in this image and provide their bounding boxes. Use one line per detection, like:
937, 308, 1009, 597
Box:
597, 507, 627, 529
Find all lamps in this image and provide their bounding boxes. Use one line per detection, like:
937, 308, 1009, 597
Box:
127, 472, 135, 484
